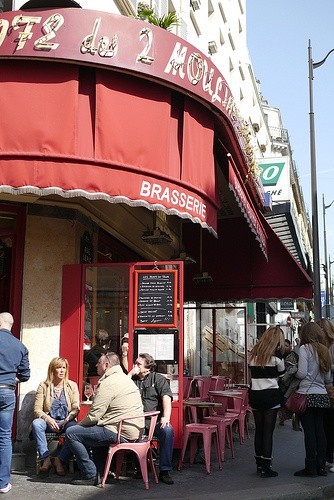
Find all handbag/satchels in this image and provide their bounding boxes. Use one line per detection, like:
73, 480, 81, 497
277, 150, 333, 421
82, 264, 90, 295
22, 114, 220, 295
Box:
285, 391, 308, 414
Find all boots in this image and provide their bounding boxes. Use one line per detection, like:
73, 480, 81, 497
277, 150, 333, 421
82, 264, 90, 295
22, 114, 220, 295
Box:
291, 419, 302, 431
295, 457, 319, 477
253, 455, 262, 472
260, 455, 278, 477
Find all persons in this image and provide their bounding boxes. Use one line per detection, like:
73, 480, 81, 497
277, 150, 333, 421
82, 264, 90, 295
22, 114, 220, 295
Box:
0, 312, 31, 494
248, 326, 286, 479
277, 318, 334, 477
124, 353, 175, 484
66, 352, 146, 485
85, 329, 129, 378
29, 357, 82, 476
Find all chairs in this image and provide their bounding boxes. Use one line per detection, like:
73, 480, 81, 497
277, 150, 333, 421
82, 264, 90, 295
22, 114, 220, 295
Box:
34, 372, 250, 491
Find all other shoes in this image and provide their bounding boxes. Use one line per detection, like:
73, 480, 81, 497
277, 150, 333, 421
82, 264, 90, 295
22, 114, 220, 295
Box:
53, 461, 65, 476
0, 483, 12, 494
134, 463, 150, 478
325, 461, 331, 466
72, 476, 96, 484
159, 472, 173, 485
39, 462, 52, 474
329, 467, 334, 473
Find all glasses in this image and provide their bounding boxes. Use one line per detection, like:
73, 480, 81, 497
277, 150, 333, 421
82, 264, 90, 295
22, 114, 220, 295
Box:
104, 351, 111, 363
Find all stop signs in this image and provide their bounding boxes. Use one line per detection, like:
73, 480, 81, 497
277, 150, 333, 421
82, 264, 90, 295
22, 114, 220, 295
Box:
287, 317, 291, 326
